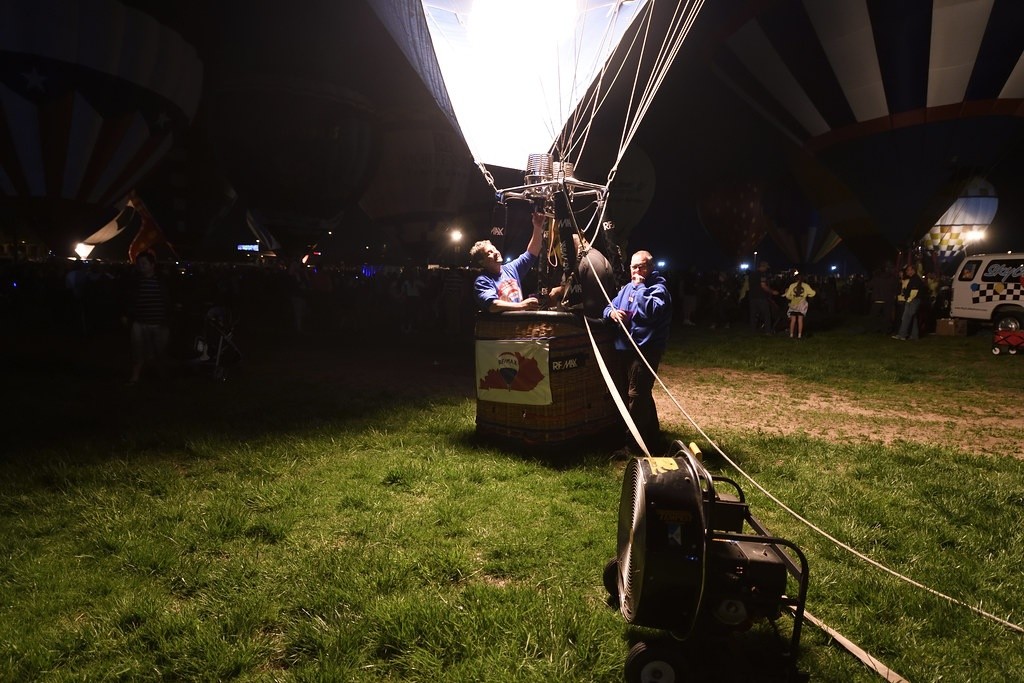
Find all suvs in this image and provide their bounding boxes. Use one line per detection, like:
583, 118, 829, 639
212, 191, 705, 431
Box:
939, 252, 1024, 334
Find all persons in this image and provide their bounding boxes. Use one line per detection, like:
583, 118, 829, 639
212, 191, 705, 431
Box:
781, 274, 816, 338
291, 262, 312, 332
739, 260, 780, 337
9, 255, 121, 340
603, 251, 673, 460
401, 262, 465, 336
680, 264, 698, 326
708, 271, 735, 329
549, 234, 618, 319
470, 212, 546, 313
122, 252, 169, 386
891, 265, 924, 340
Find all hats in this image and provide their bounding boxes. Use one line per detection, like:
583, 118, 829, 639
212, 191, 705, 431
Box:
760, 261, 771, 269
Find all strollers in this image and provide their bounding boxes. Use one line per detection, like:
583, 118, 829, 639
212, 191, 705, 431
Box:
180, 300, 246, 380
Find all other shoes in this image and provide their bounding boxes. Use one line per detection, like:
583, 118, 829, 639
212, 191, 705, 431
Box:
906, 335, 920, 340
707, 323, 716, 329
683, 318, 696, 326
891, 335, 906, 340
721, 322, 730, 328
608, 445, 638, 460
